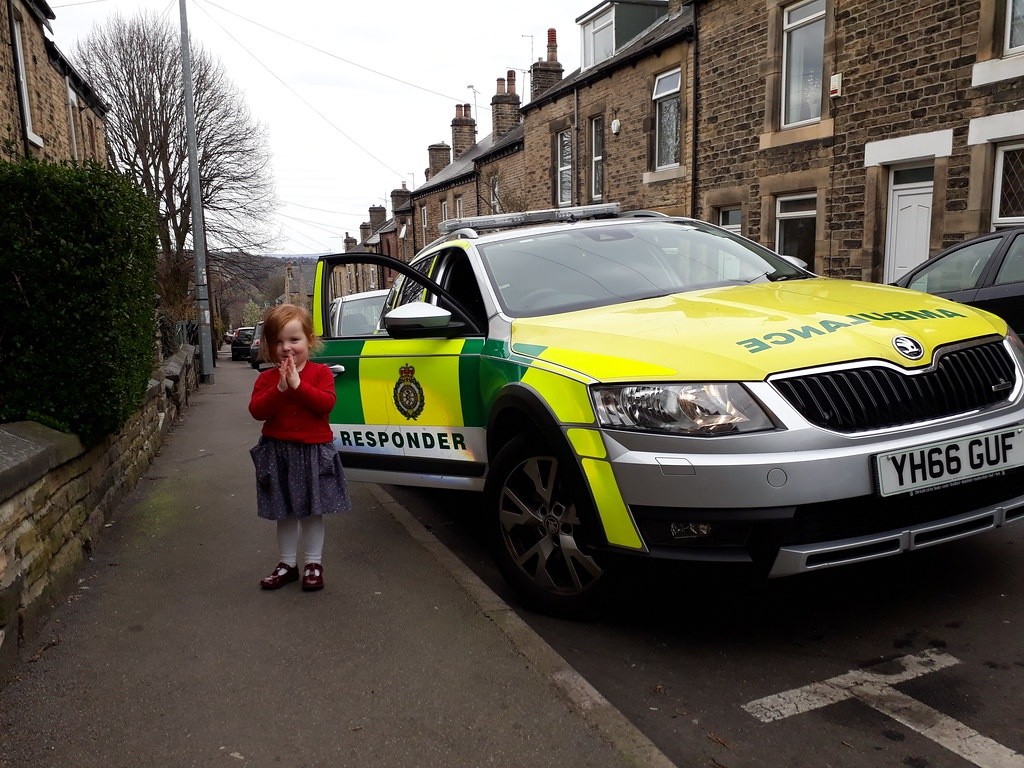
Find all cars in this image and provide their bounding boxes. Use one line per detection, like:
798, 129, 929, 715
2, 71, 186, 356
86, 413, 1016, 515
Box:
888, 227, 1024, 345
329, 289, 390, 337
226, 321, 279, 372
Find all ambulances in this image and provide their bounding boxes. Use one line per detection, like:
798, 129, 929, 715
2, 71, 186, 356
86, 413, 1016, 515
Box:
308, 202, 1024, 618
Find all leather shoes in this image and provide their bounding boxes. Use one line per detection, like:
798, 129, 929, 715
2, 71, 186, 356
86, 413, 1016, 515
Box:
301, 563, 324, 592
260, 563, 299, 589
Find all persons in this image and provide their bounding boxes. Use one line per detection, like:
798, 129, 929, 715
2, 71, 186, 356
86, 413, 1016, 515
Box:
249, 303, 352, 592
